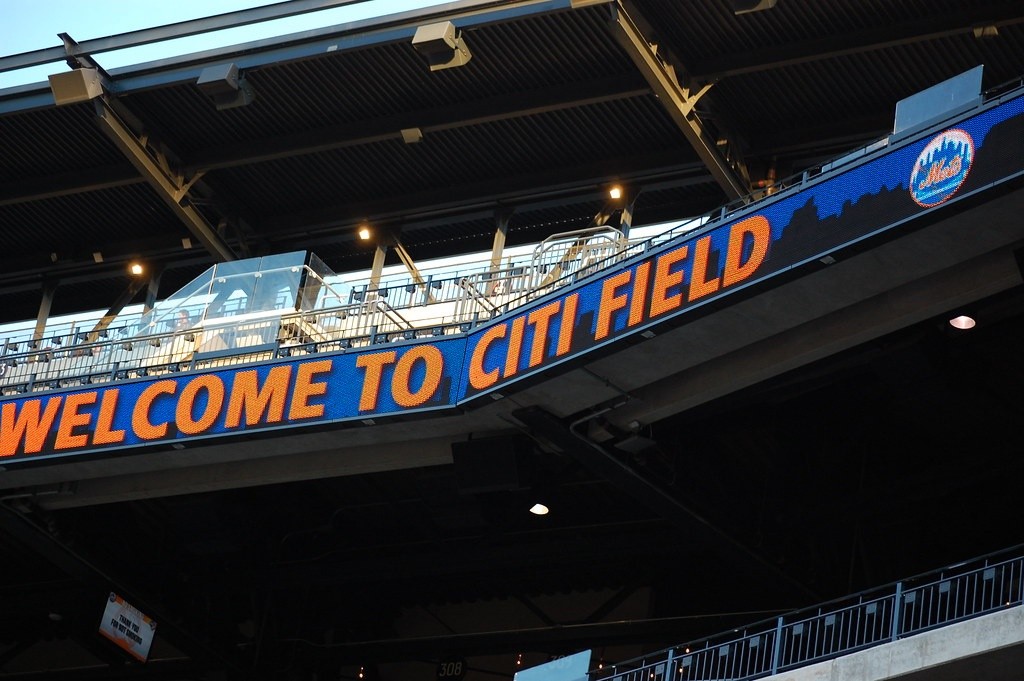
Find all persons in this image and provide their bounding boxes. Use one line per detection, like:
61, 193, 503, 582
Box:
171, 310, 190, 356
261, 296, 277, 311
